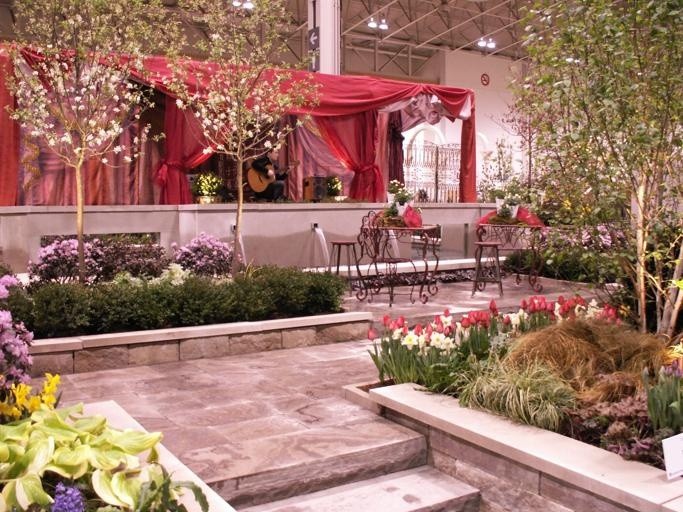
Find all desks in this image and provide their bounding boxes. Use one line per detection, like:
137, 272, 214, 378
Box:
356, 223, 441, 305
479, 221, 546, 292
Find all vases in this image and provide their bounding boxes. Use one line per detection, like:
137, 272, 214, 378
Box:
386, 191, 395, 205
507, 204, 520, 218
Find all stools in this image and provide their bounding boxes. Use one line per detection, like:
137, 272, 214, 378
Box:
329, 241, 361, 297
471, 240, 504, 297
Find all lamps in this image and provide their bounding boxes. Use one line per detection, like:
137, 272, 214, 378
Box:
367, 0, 389, 31
478, 0, 497, 49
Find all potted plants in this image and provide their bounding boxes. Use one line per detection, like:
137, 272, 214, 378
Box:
194, 170, 224, 203
493, 191, 506, 214
396, 192, 410, 216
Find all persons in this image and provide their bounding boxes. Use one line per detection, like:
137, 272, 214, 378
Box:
249, 143, 296, 202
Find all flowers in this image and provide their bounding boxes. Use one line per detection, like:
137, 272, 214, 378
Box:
388, 179, 405, 193
504, 193, 523, 205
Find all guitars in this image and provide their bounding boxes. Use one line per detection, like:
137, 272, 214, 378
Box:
247, 160, 300, 193
